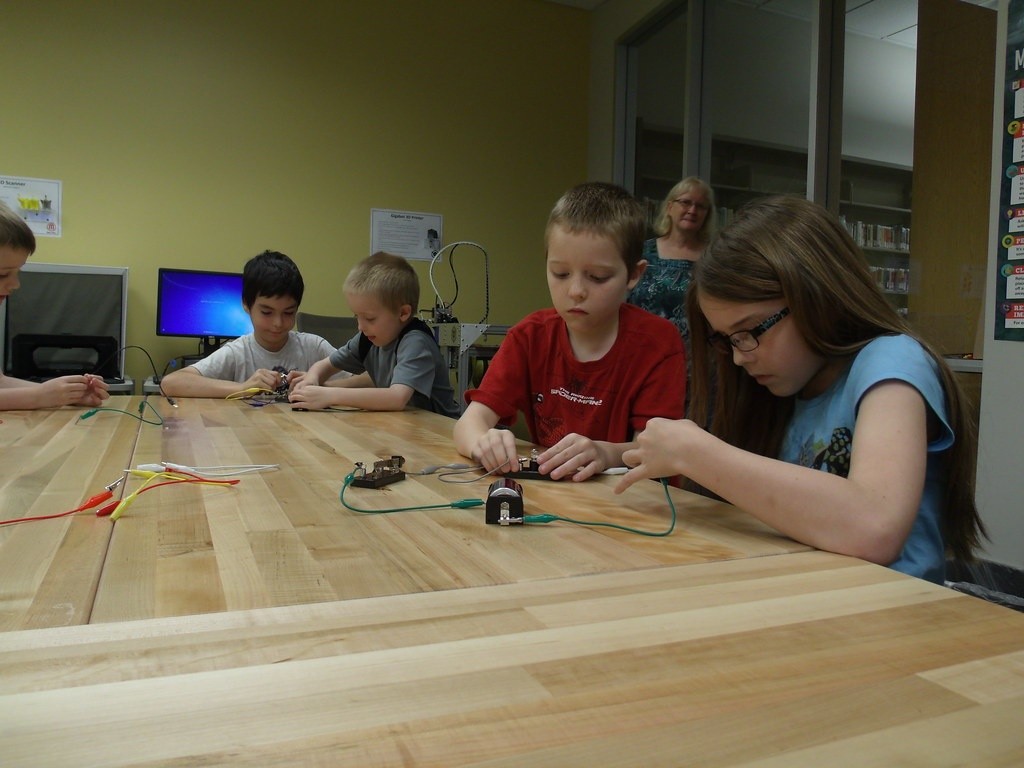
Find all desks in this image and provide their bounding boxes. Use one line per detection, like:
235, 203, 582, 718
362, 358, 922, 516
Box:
0, 396, 1024, 768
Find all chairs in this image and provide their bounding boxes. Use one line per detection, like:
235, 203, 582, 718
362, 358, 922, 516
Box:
296, 312, 358, 350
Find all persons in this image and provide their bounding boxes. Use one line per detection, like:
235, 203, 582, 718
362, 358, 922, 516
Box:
613, 196, 990, 586
629, 178, 717, 430
286, 252, 460, 419
0, 199, 110, 410
160, 251, 374, 399
454, 182, 685, 481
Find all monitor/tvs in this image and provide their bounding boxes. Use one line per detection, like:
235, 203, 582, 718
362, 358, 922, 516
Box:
155, 268, 253, 359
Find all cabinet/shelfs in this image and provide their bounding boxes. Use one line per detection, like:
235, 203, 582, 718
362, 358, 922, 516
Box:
636, 112, 911, 319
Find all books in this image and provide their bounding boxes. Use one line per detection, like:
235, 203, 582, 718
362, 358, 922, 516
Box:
838, 216, 910, 316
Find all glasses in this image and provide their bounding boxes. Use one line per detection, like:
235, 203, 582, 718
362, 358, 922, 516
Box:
707, 306, 793, 355
673, 198, 707, 209
